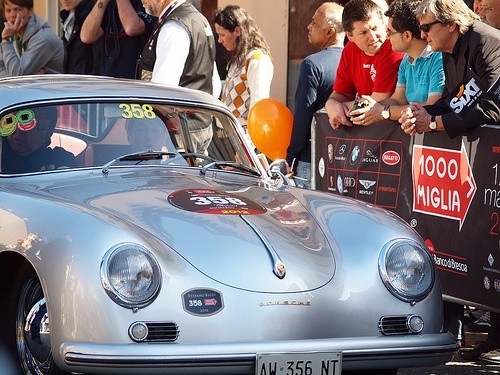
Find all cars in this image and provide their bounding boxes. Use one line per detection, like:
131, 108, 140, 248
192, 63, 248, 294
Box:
1, 72, 456, 375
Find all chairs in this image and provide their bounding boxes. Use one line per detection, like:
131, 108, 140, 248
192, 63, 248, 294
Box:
86, 142, 134, 168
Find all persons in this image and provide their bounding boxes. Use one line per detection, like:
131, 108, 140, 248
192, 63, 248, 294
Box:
347, 0, 466, 350
112, 110, 175, 166
58, 0, 96, 77
322, 1, 407, 130
79, 0, 159, 80
211, 4, 274, 155
396, 0, 500, 366
283, 2, 344, 191
135, 0, 223, 100
478, 0, 500, 31
0, 0, 65, 78
0, 105, 78, 175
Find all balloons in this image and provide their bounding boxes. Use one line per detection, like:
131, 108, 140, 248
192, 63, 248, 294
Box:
247, 98, 294, 163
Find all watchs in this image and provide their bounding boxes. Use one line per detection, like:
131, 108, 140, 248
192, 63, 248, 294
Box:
380, 105, 392, 121
429, 115, 438, 133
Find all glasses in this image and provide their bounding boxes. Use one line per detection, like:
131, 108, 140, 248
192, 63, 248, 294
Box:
418, 21, 441, 32
386, 29, 401, 36
